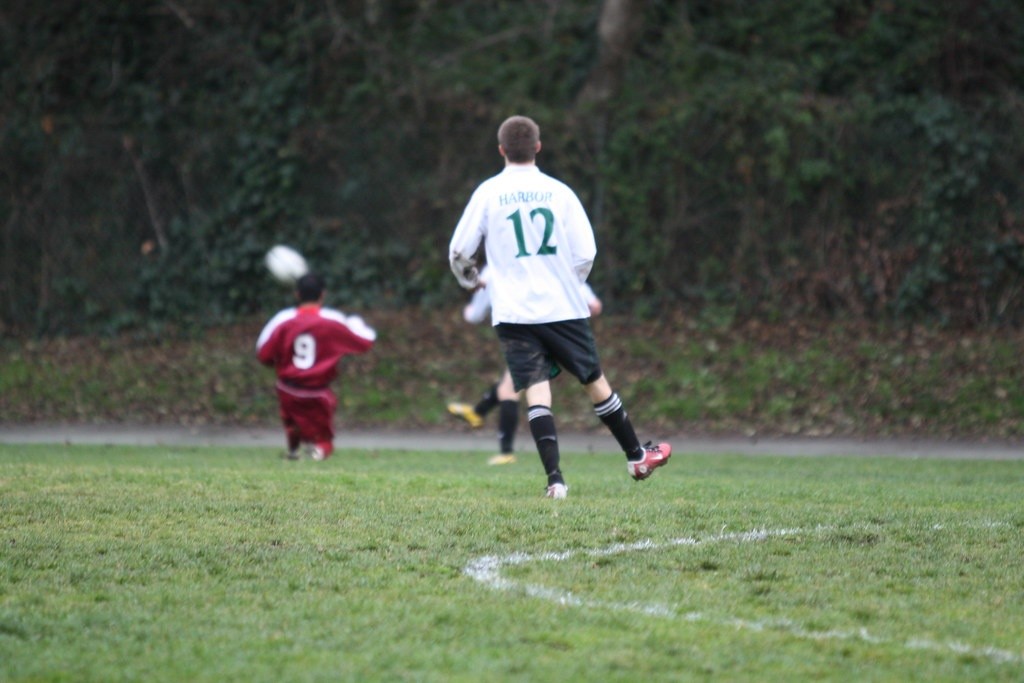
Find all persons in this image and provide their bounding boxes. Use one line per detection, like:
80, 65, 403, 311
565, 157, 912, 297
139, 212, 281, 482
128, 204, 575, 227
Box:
255, 273, 376, 460
448, 115, 671, 501
449, 262, 603, 466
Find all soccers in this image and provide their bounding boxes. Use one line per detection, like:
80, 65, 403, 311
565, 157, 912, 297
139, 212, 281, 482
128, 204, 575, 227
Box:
264, 243, 310, 282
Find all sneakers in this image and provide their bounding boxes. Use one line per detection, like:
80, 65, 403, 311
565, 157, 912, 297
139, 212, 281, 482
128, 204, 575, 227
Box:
545, 485, 568, 500
448, 400, 483, 428
627, 440, 672, 481
487, 453, 515, 467
